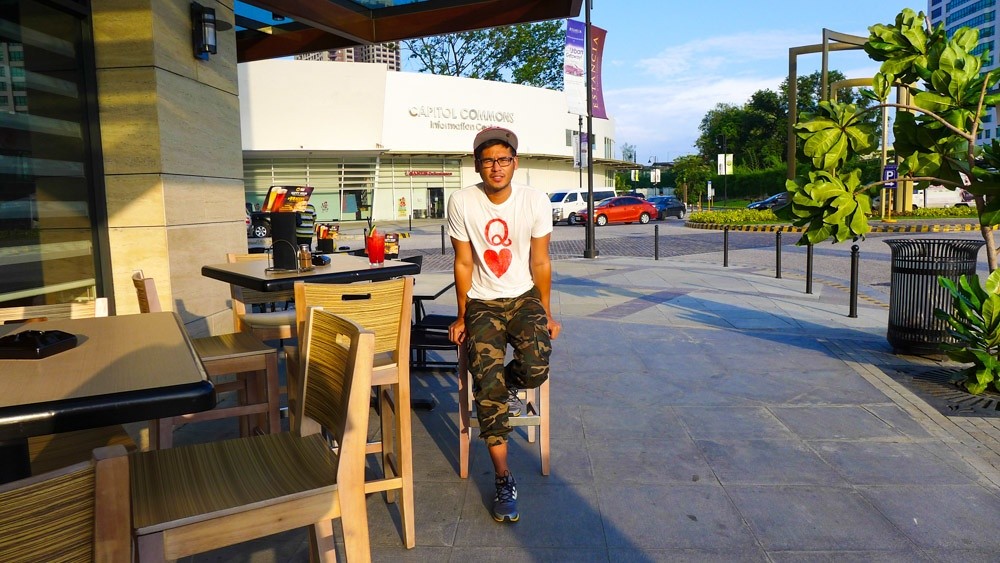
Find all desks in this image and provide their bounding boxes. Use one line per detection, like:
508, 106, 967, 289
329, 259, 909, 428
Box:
202, 254, 421, 300
0, 312, 218, 490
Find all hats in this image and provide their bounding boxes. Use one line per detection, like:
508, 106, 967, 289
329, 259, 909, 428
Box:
473, 125, 519, 153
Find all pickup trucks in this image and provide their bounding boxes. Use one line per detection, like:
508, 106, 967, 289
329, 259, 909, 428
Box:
246, 202, 301, 238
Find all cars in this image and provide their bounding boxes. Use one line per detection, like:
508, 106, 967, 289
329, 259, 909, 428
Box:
646, 195, 686, 221
575, 195, 659, 225
747, 191, 791, 211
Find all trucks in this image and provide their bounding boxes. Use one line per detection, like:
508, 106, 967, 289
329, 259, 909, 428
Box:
871, 172, 988, 211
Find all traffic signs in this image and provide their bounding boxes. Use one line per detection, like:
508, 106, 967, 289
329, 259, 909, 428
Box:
884, 168, 898, 188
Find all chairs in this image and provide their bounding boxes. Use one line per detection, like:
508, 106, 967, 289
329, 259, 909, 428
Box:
130, 269, 282, 449
457, 343, 551, 479
0, 445, 130, 563
285, 277, 417, 550
131, 306, 375, 563
227, 253, 300, 441
0, 298, 138, 477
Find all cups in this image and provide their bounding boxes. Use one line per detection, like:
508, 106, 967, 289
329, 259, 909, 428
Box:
317, 239, 333, 254
367, 236, 385, 266
266, 187, 287, 212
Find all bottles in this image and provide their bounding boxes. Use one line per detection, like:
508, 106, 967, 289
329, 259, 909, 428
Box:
315, 219, 339, 251
384, 236, 399, 260
299, 244, 312, 270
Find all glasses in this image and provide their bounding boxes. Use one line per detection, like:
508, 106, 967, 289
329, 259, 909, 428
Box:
477, 157, 514, 167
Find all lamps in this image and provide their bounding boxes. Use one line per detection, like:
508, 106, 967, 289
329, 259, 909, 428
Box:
190, 1, 232, 61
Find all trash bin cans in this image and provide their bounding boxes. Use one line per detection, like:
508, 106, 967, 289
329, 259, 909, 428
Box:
882, 238, 986, 357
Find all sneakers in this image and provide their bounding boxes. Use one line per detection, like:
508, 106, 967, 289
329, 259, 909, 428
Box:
491, 473, 520, 522
506, 386, 523, 417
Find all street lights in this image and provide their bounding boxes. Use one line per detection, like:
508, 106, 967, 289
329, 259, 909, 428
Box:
648, 155, 658, 196
715, 132, 728, 203
628, 150, 638, 195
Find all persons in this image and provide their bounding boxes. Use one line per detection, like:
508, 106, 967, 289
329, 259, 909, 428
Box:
447, 126, 561, 526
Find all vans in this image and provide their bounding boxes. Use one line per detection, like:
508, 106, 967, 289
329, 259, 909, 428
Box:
548, 186, 617, 228
